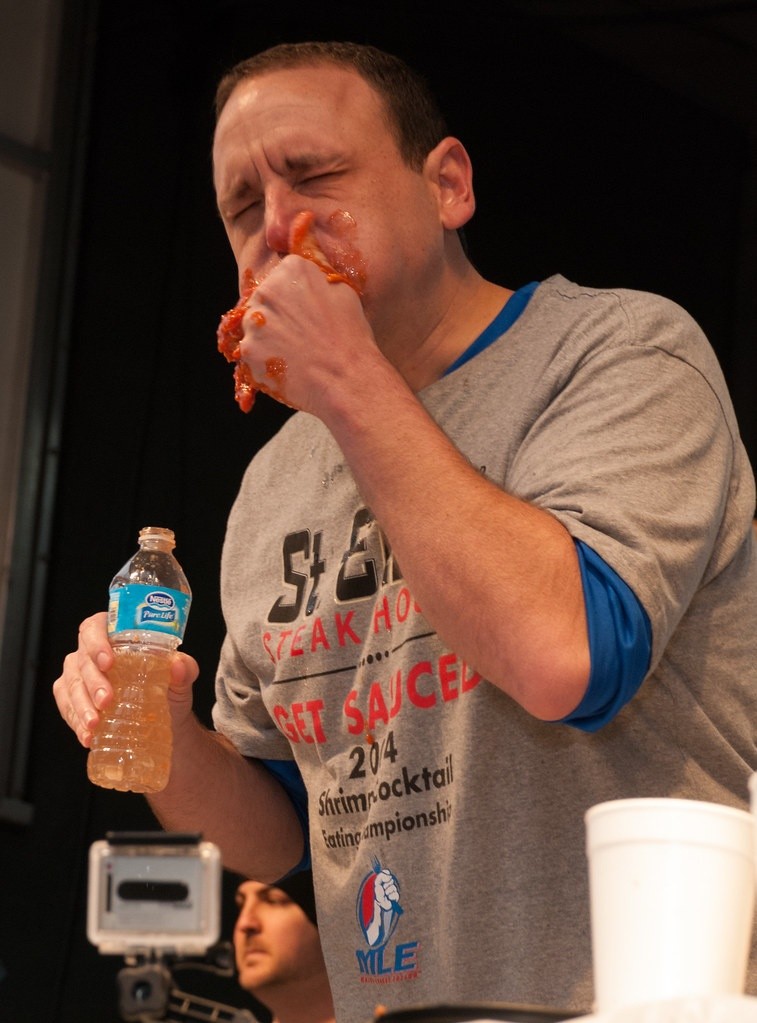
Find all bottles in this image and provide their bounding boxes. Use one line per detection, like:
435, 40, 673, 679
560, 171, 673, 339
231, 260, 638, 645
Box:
84, 526, 191, 793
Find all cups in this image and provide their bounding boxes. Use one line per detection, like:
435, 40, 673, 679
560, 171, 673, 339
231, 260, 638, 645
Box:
581, 798, 757, 1023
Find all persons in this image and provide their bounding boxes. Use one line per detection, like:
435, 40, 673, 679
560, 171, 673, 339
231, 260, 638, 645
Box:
51, 42, 757, 1023
233, 879, 351, 1023
